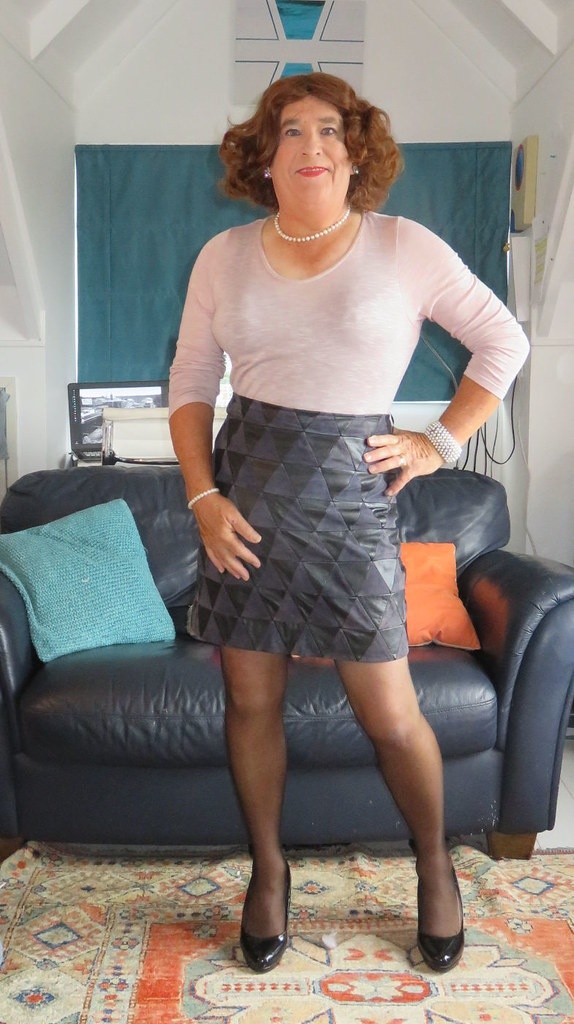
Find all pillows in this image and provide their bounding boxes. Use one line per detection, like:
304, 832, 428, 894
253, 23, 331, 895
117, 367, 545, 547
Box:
398, 542, 481, 652
0, 497, 176, 662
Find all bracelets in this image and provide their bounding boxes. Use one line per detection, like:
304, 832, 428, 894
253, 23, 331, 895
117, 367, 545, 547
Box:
188, 489, 222, 510
424, 421, 462, 464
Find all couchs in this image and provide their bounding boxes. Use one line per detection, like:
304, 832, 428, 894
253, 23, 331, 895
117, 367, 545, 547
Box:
0, 470, 574, 860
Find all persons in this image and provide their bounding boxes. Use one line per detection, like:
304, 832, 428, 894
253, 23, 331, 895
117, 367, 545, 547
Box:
166, 72, 531, 972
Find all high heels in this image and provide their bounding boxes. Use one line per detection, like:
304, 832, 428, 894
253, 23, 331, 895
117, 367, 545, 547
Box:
239, 857, 291, 973
408, 839, 465, 973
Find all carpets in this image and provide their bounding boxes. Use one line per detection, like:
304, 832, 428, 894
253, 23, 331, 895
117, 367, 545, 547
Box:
0, 840, 574, 1024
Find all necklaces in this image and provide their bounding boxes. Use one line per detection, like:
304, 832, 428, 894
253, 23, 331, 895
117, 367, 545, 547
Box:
274, 203, 351, 242
